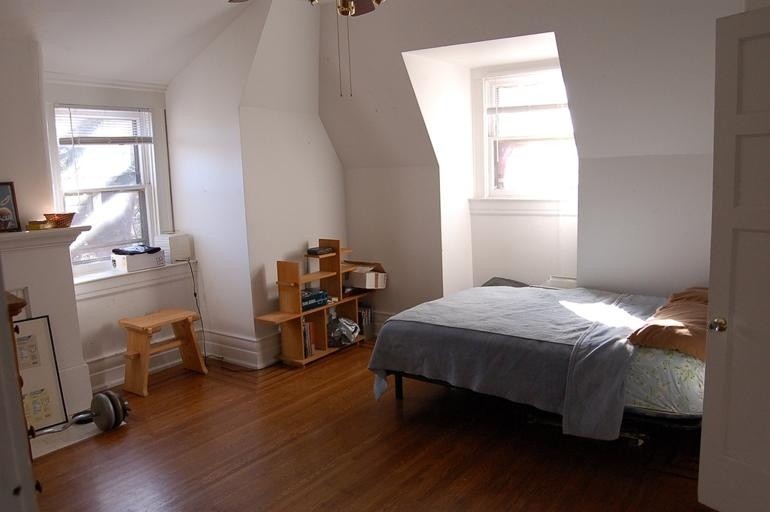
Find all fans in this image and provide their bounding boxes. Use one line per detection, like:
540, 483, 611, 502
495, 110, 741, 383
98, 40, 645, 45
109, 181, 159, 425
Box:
228, 1, 388, 20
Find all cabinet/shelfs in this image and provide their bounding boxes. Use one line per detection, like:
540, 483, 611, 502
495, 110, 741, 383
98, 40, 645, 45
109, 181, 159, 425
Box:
253, 237, 378, 369
3, 288, 43, 496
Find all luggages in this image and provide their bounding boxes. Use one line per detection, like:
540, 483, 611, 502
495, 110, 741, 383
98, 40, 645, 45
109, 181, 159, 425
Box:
510, 400, 675, 463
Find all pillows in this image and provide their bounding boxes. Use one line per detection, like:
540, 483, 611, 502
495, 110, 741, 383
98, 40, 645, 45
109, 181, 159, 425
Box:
672, 285, 708, 307
629, 302, 707, 362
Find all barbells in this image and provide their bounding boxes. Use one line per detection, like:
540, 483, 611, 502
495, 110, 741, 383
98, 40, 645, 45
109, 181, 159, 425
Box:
25, 389, 129, 437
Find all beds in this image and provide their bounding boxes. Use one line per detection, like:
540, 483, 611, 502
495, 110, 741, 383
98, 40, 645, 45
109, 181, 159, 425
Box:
363, 274, 715, 445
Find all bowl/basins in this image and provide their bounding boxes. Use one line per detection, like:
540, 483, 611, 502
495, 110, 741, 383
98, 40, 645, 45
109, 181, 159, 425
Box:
44, 213, 75, 227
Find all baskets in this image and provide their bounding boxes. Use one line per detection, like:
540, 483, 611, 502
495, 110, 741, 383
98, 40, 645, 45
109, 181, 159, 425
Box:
44, 214, 74, 228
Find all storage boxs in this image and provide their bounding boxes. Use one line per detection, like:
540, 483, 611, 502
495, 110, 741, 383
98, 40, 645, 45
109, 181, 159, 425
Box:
340, 260, 389, 292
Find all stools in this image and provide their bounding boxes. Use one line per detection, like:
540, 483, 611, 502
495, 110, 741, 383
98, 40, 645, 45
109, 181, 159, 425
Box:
117, 306, 210, 399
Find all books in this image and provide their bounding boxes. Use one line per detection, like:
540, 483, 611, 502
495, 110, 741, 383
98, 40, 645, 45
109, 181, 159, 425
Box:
153, 228, 194, 266
300, 302, 373, 360
